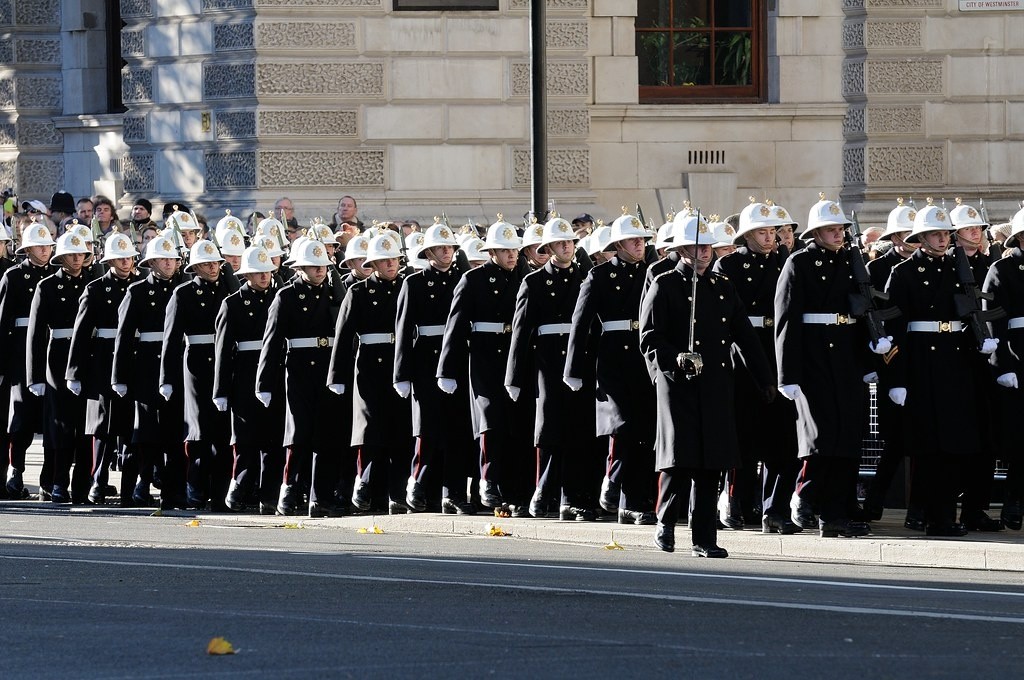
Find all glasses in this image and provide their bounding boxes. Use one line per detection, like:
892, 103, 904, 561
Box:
275, 206, 292, 212
25, 208, 41, 213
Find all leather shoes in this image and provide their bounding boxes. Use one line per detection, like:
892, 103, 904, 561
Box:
0, 464, 1024, 558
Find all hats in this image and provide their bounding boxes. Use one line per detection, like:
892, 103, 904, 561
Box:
163, 203, 189, 218
21, 200, 47, 214
133, 198, 152, 215
572, 214, 595, 224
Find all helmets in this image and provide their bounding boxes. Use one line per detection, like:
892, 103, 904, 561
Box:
0, 190, 1024, 274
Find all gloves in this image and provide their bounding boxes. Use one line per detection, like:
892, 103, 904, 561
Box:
329, 384, 345, 394
976, 338, 999, 354
213, 397, 228, 411
255, 391, 271, 408
159, 384, 172, 401
505, 385, 520, 401
563, 376, 582, 391
29, 383, 46, 396
393, 381, 410, 398
754, 384, 776, 404
684, 359, 704, 375
777, 336, 1018, 406
438, 377, 457, 394
112, 383, 127, 397
66, 380, 81, 395
475, 395, 490, 411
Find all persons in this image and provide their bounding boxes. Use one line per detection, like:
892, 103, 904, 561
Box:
0, 190, 1024, 558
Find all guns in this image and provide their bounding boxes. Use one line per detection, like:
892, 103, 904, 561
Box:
635, 202, 659, 265
776, 233, 790, 262
850, 208, 870, 264
978, 197, 1002, 262
308, 216, 347, 305
909, 195, 919, 212
170, 209, 200, 283
261, 208, 289, 290
90, 220, 138, 279
575, 245, 595, 272
940, 196, 1006, 351
441, 209, 487, 278
834, 198, 902, 349
207, 210, 257, 296
398, 224, 415, 279
10, 213, 48, 263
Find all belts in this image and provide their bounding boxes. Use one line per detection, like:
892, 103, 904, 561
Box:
15, 318, 639, 349
747, 313, 1024, 333
